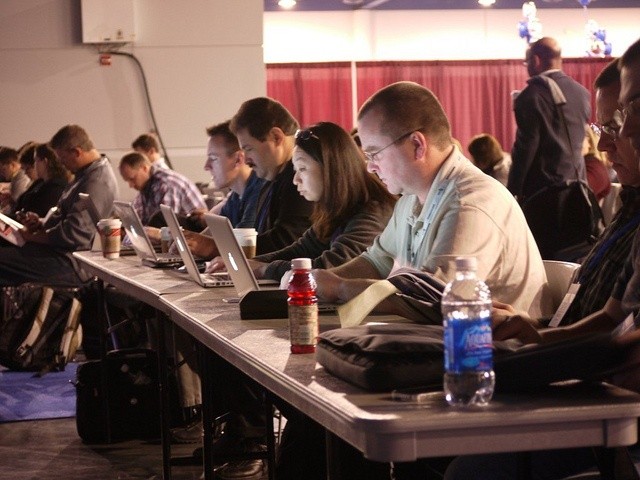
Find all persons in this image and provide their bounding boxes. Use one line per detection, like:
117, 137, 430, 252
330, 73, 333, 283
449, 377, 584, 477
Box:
118, 152, 210, 250
182, 96, 302, 479
205, 120, 399, 480
3, 142, 43, 221
0, 124, 115, 287
165, 118, 273, 444
508, 36, 593, 264
465, 130, 514, 188
11, 141, 74, 226
441, 34, 640, 479
581, 107, 612, 201
131, 135, 172, 172
0, 147, 32, 213
469, 54, 640, 343
271, 79, 557, 480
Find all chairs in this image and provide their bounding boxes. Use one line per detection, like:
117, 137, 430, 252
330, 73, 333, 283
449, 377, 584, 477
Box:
538, 259, 582, 331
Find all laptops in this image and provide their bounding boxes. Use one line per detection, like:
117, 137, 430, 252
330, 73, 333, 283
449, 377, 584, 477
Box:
112, 198, 204, 264
159, 204, 280, 291
202, 212, 342, 313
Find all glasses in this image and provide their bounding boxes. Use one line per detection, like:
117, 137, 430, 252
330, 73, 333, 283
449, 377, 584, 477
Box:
363, 128, 423, 161
294, 129, 318, 143
590, 122, 622, 139
617, 96, 639, 123
521, 58, 530, 66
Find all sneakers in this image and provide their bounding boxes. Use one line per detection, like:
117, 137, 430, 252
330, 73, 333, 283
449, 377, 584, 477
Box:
193, 429, 268, 462
169, 418, 225, 443
219, 446, 273, 480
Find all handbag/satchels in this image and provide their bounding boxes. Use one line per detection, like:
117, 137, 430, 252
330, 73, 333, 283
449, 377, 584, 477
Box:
525, 180, 605, 245
69, 347, 161, 445
315, 323, 548, 394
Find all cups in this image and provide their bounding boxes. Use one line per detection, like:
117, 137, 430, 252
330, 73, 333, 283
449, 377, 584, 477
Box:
232, 227, 259, 259
96, 218, 122, 259
160, 226, 184, 253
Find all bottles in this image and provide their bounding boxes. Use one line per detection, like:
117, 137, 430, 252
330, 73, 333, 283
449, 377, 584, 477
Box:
439, 255, 497, 408
287, 258, 319, 354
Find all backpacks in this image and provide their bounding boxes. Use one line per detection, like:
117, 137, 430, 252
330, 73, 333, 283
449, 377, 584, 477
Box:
0, 283, 82, 378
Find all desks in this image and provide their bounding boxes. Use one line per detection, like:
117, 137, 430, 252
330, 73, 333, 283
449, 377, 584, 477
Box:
69, 232, 639, 479
